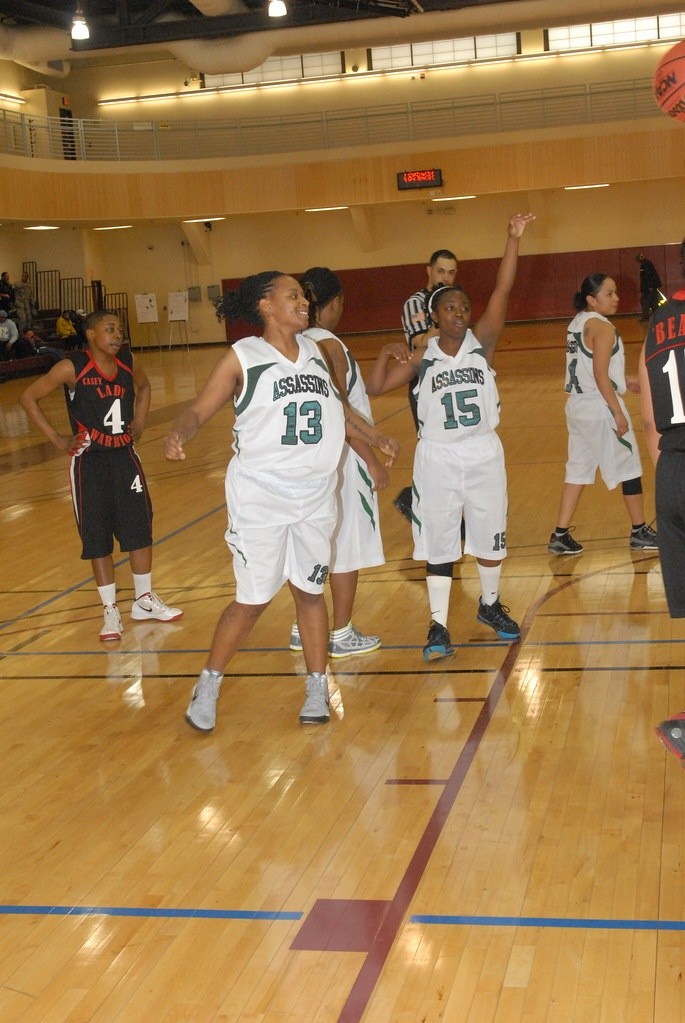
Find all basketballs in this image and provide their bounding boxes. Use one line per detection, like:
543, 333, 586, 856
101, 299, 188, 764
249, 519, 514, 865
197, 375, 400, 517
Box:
651, 38, 685, 123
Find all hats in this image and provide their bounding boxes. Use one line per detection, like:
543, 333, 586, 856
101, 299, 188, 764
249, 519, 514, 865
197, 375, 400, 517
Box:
0, 310, 8, 318
76, 308, 87, 317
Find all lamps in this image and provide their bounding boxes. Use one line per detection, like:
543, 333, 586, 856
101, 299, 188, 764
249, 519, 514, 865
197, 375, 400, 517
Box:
268, 0, 288, 17
70, 0, 90, 40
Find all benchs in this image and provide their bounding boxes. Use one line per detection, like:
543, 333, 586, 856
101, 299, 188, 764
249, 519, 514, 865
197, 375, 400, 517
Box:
0, 302, 130, 380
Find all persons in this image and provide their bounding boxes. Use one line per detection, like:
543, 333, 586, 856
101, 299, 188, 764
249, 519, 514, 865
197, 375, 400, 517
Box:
636, 252, 662, 323
0, 272, 18, 319
638, 236, 685, 761
547, 274, 659, 555
0, 307, 48, 361
393, 249, 465, 542
19, 312, 184, 641
55, 310, 86, 350
289, 267, 390, 658
161, 271, 402, 732
366, 214, 536, 660
13, 271, 38, 333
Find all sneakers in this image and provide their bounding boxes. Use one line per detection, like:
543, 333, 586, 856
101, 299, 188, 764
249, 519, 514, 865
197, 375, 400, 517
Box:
133, 619, 183, 645
476, 594, 521, 639
629, 518, 659, 551
101, 640, 123, 651
421, 619, 454, 662
327, 619, 383, 658
185, 666, 225, 732
298, 672, 331, 723
548, 526, 584, 555
655, 713, 685, 764
289, 619, 303, 651
98, 603, 124, 641
130, 591, 184, 622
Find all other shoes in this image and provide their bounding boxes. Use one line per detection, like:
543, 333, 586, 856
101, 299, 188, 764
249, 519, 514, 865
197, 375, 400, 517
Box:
392, 499, 413, 523
639, 317, 650, 323
